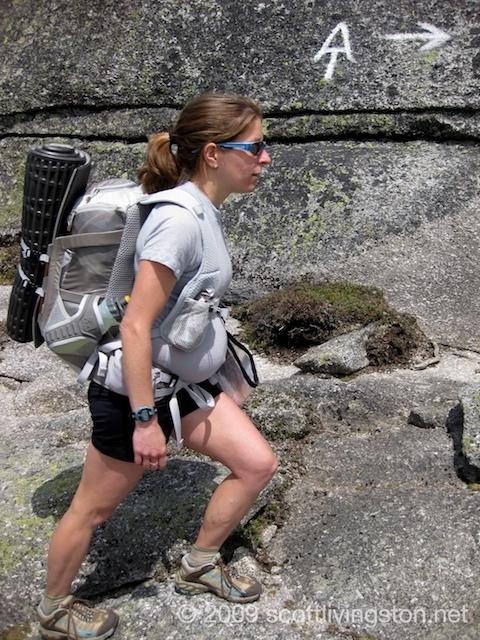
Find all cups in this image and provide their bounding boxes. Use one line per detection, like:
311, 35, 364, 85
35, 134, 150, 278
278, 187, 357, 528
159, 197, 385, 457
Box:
49, 296, 129, 355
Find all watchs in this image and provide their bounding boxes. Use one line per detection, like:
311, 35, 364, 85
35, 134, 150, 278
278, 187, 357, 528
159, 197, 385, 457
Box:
132, 407, 158, 422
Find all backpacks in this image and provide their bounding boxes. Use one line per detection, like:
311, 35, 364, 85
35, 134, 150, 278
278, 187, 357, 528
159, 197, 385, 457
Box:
36, 178, 203, 384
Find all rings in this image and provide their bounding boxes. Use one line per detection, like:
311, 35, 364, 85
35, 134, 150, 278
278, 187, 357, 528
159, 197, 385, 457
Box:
150, 462, 158, 466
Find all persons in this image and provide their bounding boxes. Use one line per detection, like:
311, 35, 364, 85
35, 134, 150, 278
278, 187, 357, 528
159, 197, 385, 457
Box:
37, 92, 278, 640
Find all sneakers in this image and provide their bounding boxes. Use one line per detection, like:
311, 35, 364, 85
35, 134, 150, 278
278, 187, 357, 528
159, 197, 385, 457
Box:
37, 595, 119, 640
175, 553, 262, 604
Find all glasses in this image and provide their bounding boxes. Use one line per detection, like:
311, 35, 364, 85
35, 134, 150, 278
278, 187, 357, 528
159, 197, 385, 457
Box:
217, 141, 267, 158
